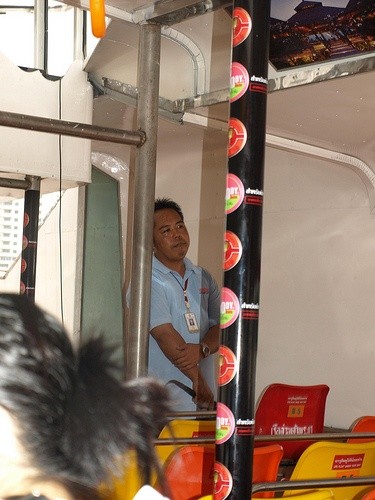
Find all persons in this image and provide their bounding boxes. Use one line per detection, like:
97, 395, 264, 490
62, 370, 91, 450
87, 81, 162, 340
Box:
1, 292, 158, 498
149, 197, 222, 422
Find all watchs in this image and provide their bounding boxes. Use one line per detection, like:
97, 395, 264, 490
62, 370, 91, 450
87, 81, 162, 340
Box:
199, 343, 210, 358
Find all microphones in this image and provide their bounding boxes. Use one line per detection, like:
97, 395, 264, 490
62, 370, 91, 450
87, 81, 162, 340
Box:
177, 383, 217, 412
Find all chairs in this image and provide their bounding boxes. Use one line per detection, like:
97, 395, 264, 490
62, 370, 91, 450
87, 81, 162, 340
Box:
281, 439, 375, 499
255, 382, 335, 466
159, 446, 214, 500
346, 417, 375, 444
151, 419, 216, 483
